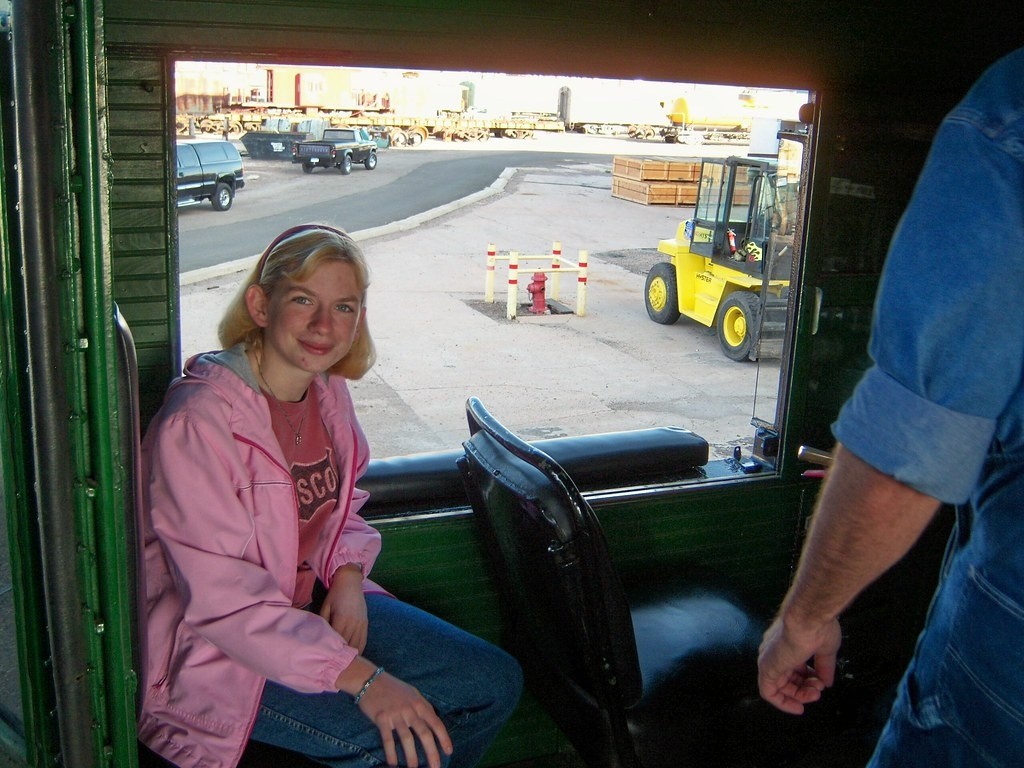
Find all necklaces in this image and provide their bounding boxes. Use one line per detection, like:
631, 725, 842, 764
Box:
253, 349, 312, 444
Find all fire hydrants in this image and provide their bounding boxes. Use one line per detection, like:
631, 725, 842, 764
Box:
526, 268, 548, 313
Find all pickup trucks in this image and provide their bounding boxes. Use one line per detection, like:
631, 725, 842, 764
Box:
177, 141, 245, 211
291, 128, 378, 175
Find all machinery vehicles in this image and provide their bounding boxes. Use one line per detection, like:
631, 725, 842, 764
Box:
659, 97, 768, 144
644, 155, 801, 362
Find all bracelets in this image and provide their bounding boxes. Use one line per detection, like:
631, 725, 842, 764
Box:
354, 667, 385, 704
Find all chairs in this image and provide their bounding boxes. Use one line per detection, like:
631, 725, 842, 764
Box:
455, 396, 764, 768
114, 300, 328, 767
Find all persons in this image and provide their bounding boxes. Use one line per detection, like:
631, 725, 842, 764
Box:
139, 219, 525, 768
754, 37, 1024, 768
